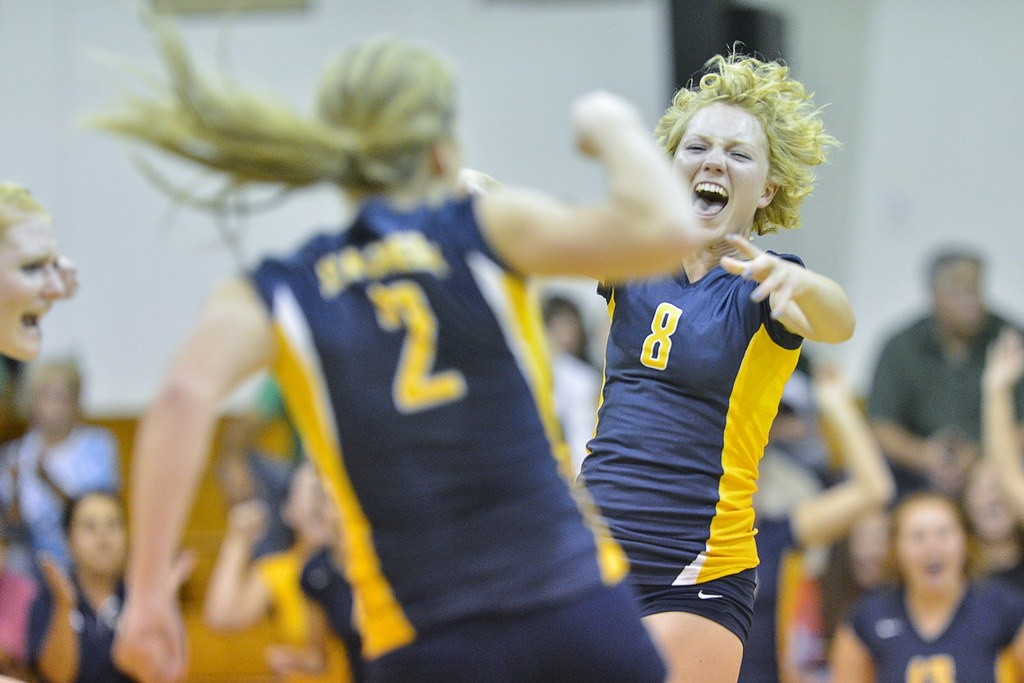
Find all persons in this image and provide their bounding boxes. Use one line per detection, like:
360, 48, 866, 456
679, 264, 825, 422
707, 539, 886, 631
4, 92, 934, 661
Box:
203, 464, 365, 683
821, 489, 1024, 683
113, 39, 712, 683
3, 185, 75, 398
0, 365, 122, 590
24, 487, 182, 683
546, 257, 1024, 683
574, 37, 856, 683
